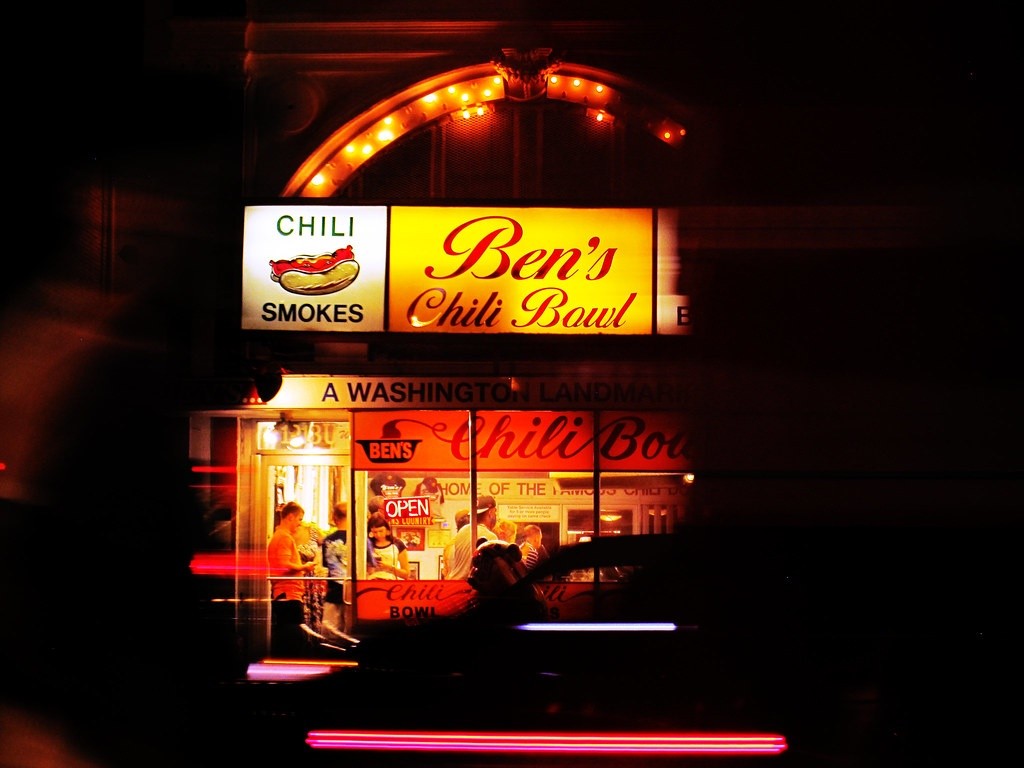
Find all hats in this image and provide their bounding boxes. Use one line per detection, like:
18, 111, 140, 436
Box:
469, 496, 495, 515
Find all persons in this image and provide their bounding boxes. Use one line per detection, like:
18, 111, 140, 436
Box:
268, 494, 543, 659
